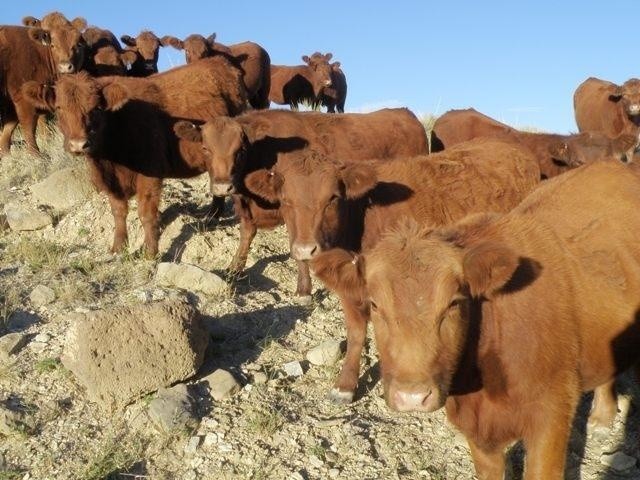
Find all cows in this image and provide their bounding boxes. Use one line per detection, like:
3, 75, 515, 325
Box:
574, 77, 640, 139
0, 1, 346, 113
428, 215, 431, 217
172, 106, 431, 307
244, 134, 541, 405
427, 108, 639, 179
2, 17, 88, 161
53, 58, 245, 260
308, 159, 640, 480
